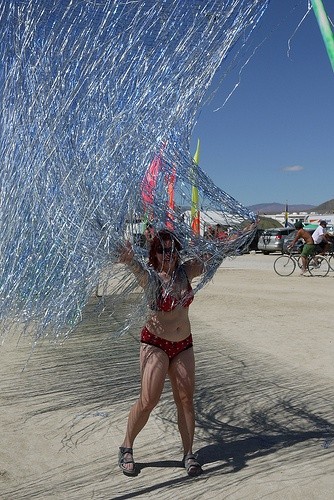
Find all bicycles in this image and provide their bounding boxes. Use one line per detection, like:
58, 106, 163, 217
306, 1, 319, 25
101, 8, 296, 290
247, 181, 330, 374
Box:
274, 244, 334, 277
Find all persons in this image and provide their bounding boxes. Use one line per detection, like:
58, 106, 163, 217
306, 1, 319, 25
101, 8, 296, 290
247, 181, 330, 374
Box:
124, 216, 244, 256
287, 222, 315, 275
311, 221, 334, 267
114, 223, 257, 477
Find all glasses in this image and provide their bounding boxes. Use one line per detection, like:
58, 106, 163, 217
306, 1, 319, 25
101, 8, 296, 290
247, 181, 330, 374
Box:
157, 246, 176, 254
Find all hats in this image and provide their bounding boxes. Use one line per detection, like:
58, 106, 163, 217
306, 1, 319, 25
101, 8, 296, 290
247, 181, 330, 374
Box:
319, 221, 327, 225
294, 221, 304, 228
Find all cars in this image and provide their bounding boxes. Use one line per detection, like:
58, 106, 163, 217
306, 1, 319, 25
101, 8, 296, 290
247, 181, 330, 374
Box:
257, 214, 334, 255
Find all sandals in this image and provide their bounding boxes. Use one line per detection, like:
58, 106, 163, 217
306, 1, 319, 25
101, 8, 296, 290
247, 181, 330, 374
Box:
118, 447, 136, 474
183, 454, 202, 478
